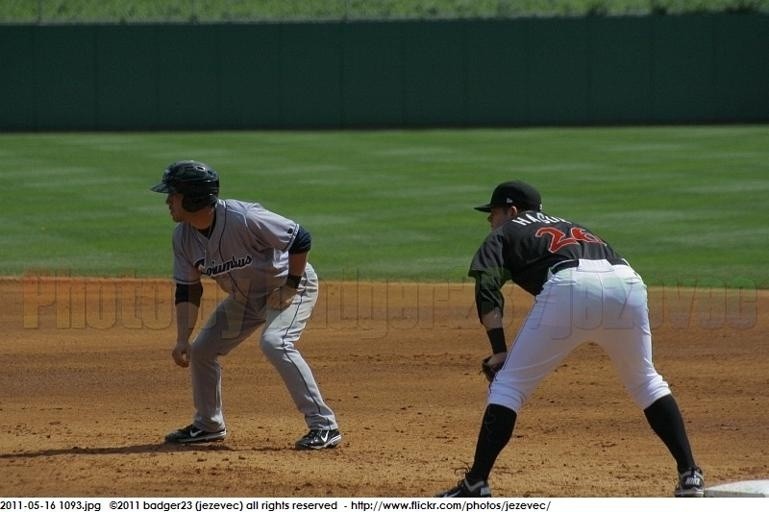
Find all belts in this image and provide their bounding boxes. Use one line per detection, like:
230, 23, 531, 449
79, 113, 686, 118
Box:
551, 257, 628, 273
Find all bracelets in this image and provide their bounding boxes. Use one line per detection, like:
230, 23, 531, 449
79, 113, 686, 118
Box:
284, 273, 303, 289
486, 326, 507, 356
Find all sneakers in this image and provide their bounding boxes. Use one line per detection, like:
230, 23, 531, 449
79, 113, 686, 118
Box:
433, 472, 491, 497
295, 429, 341, 449
165, 423, 226, 443
674, 466, 705, 497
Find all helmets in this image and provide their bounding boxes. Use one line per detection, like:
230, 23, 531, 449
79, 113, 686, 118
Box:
149, 159, 218, 194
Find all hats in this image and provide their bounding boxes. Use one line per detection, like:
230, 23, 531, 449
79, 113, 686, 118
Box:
473, 181, 542, 212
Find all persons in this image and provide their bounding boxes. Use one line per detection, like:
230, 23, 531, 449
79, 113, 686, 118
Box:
431, 176, 709, 498
148, 157, 342, 451
